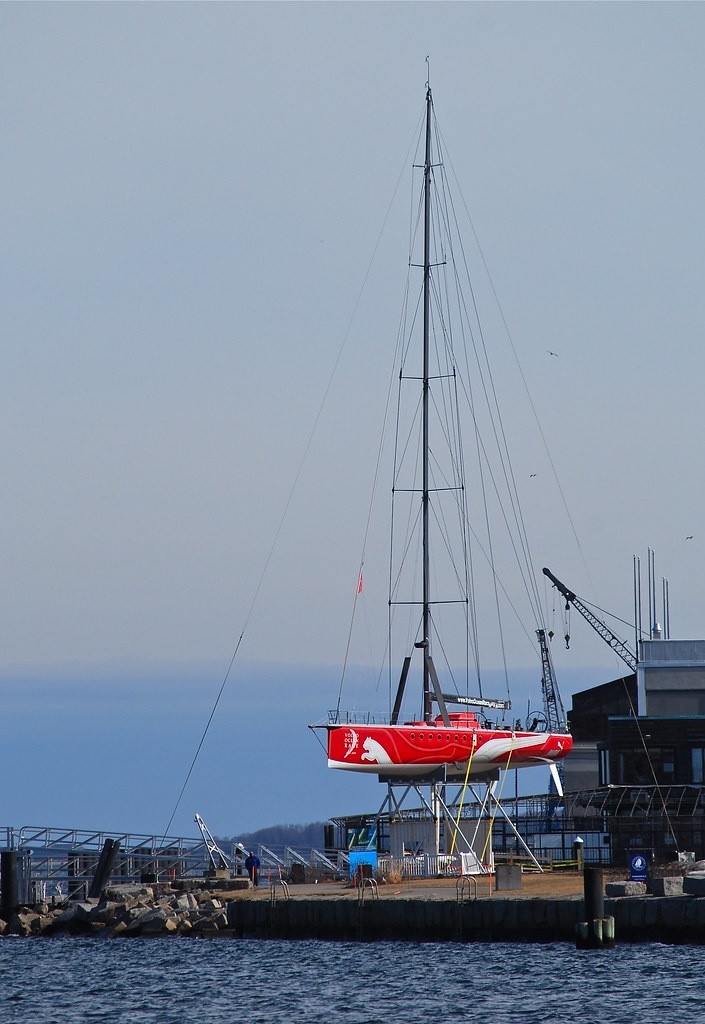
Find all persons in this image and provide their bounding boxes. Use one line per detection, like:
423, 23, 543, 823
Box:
245, 852, 260, 886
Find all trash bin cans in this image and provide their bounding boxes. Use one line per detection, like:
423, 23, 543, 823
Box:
625, 847, 654, 882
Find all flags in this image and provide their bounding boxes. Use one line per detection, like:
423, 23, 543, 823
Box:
359, 568, 364, 593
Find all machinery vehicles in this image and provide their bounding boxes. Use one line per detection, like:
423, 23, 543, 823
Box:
540, 566, 638, 673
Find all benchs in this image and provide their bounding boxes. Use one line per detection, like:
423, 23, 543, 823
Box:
461, 852, 495, 875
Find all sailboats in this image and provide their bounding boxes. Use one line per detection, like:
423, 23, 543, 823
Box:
305, 55, 577, 779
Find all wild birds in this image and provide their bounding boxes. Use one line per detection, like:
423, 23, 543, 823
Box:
530, 474, 536, 477
546, 350, 559, 357
686, 536, 693, 540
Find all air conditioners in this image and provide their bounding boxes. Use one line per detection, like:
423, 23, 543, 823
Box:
631, 792, 647, 802
